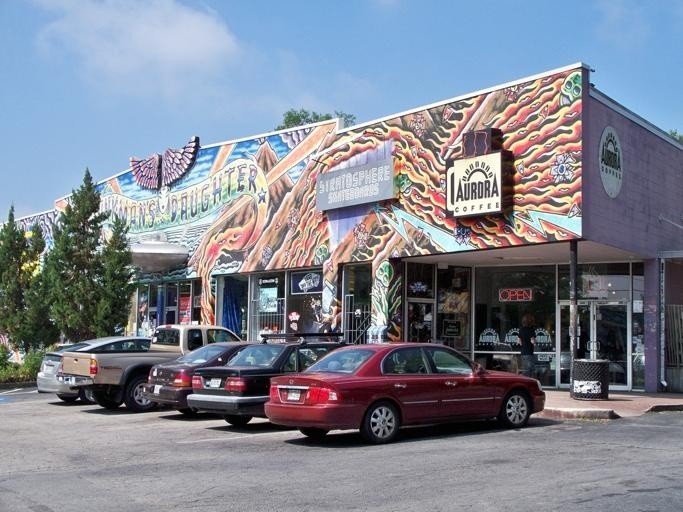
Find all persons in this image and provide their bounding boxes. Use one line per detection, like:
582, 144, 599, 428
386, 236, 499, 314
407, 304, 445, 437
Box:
517, 312, 537, 378
317, 315, 337, 343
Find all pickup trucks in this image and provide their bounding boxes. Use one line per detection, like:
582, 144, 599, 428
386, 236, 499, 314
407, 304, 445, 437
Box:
56, 324, 244, 413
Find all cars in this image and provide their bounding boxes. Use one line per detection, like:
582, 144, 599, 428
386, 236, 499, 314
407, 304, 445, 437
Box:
141, 341, 254, 415
187, 338, 350, 427
36, 337, 153, 404
264, 342, 547, 444
